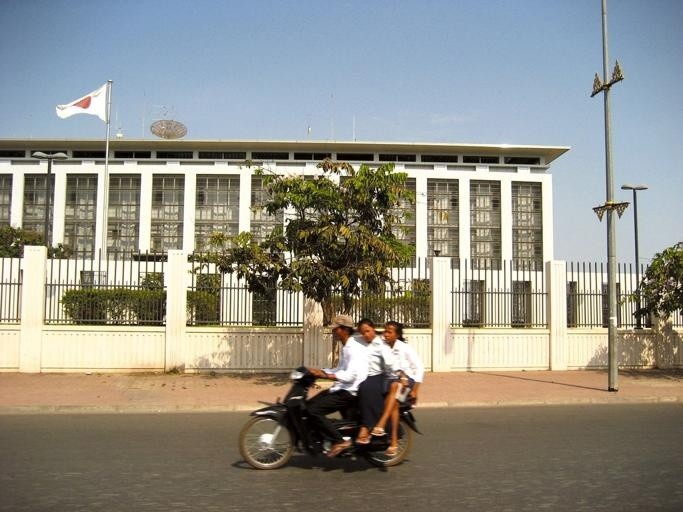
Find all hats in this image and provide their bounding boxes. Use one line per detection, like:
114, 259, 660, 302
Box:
327, 315, 353, 329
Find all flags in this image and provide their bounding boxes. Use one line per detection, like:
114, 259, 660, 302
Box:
56, 82, 109, 121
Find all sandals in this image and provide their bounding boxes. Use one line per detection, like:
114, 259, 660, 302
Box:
372, 427, 388, 437
329, 441, 352, 457
356, 433, 372, 444
385, 446, 399, 457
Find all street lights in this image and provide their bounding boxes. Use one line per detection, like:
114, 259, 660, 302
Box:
621, 184, 648, 330
32, 151, 67, 247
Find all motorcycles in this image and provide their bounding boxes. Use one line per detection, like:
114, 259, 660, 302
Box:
238, 366, 416, 470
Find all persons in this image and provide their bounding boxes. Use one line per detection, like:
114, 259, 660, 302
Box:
356, 319, 399, 444
310, 313, 366, 459
370, 321, 425, 457
